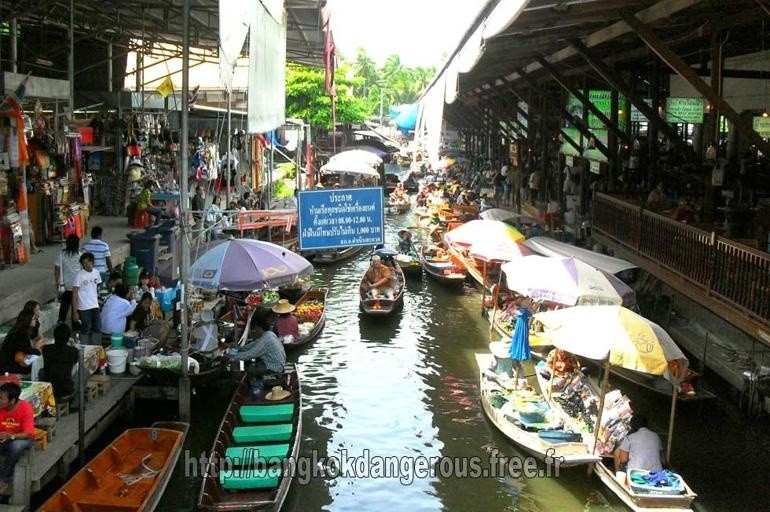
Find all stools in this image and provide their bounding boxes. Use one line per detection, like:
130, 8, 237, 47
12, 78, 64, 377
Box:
56, 402, 70, 421
34, 417, 57, 442
90, 374, 112, 396
34, 428, 49, 451
20, 444, 36, 465
85, 380, 99, 404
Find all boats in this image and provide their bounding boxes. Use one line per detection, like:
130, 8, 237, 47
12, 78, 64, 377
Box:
269, 285, 331, 349
533, 361, 645, 462
198, 362, 301, 511
318, 217, 377, 263
595, 449, 697, 512
133, 300, 256, 392
474, 351, 602, 469
39, 422, 187, 512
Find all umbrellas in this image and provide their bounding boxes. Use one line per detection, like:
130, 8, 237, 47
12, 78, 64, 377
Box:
499, 256, 637, 305
533, 305, 689, 473
509, 308, 531, 389
446, 217, 526, 246
469, 237, 535, 263
182, 238, 314, 346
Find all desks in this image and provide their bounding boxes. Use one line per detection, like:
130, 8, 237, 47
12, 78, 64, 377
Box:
19, 380, 57, 418
30, 340, 107, 381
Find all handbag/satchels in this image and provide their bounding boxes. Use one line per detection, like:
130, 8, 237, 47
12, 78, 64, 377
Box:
57, 283, 66, 301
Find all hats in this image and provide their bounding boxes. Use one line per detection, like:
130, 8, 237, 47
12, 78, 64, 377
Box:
127, 166, 142, 182
371, 255, 381, 261
200, 307, 215, 323
265, 386, 292, 401
128, 159, 143, 166
272, 299, 296, 314
398, 230, 412, 239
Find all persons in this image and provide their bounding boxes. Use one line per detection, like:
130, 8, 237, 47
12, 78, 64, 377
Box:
192, 185, 301, 239
137, 181, 162, 227
0, 227, 298, 496
496, 146, 713, 230
486, 336, 522, 388
546, 346, 587, 393
323, 137, 498, 223
613, 416, 664, 473
365, 223, 448, 298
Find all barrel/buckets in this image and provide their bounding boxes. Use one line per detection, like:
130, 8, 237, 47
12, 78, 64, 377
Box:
107, 350, 128, 374
